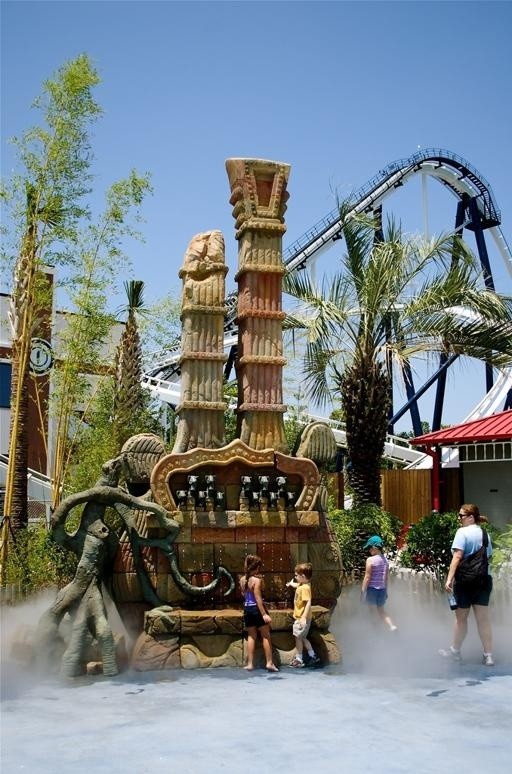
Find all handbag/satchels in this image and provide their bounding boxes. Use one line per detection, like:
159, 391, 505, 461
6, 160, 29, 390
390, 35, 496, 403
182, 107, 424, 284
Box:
455, 548, 488, 585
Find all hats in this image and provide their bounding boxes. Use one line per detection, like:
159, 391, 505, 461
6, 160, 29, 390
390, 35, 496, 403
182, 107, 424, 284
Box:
364, 536, 383, 550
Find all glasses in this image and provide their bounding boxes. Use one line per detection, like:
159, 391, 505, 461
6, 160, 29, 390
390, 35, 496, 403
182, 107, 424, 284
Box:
459, 514, 469, 518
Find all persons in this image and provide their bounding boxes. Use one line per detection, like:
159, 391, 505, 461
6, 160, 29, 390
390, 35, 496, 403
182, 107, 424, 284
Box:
361, 535, 398, 636
285, 563, 320, 668
240, 554, 280, 672
437, 503, 495, 667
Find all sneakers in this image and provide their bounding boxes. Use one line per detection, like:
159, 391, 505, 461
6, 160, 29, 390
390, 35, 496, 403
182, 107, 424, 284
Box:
482, 653, 493, 666
305, 654, 320, 666
289, 659, 305, 668
438, 647, 461, 661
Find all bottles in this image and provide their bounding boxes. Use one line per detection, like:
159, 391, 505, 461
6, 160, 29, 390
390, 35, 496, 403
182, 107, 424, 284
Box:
449, 591, 459, 611
